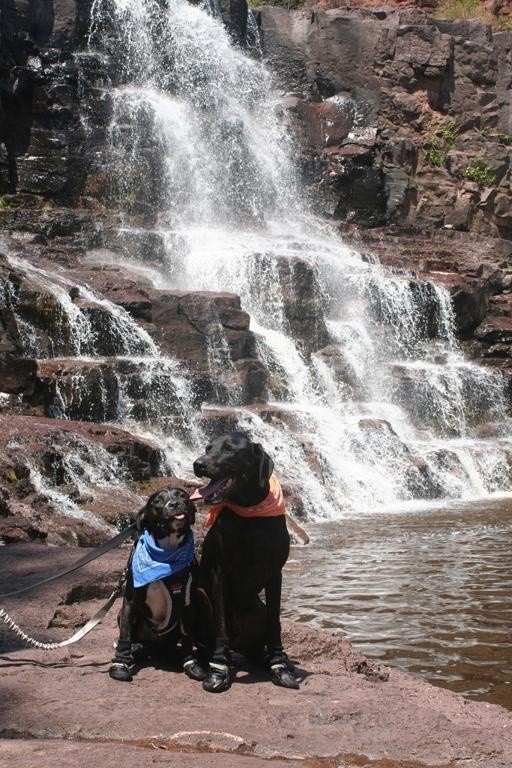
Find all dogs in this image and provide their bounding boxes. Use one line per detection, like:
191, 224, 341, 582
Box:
188, 430, 300, 693
108, 487, 215, 682
